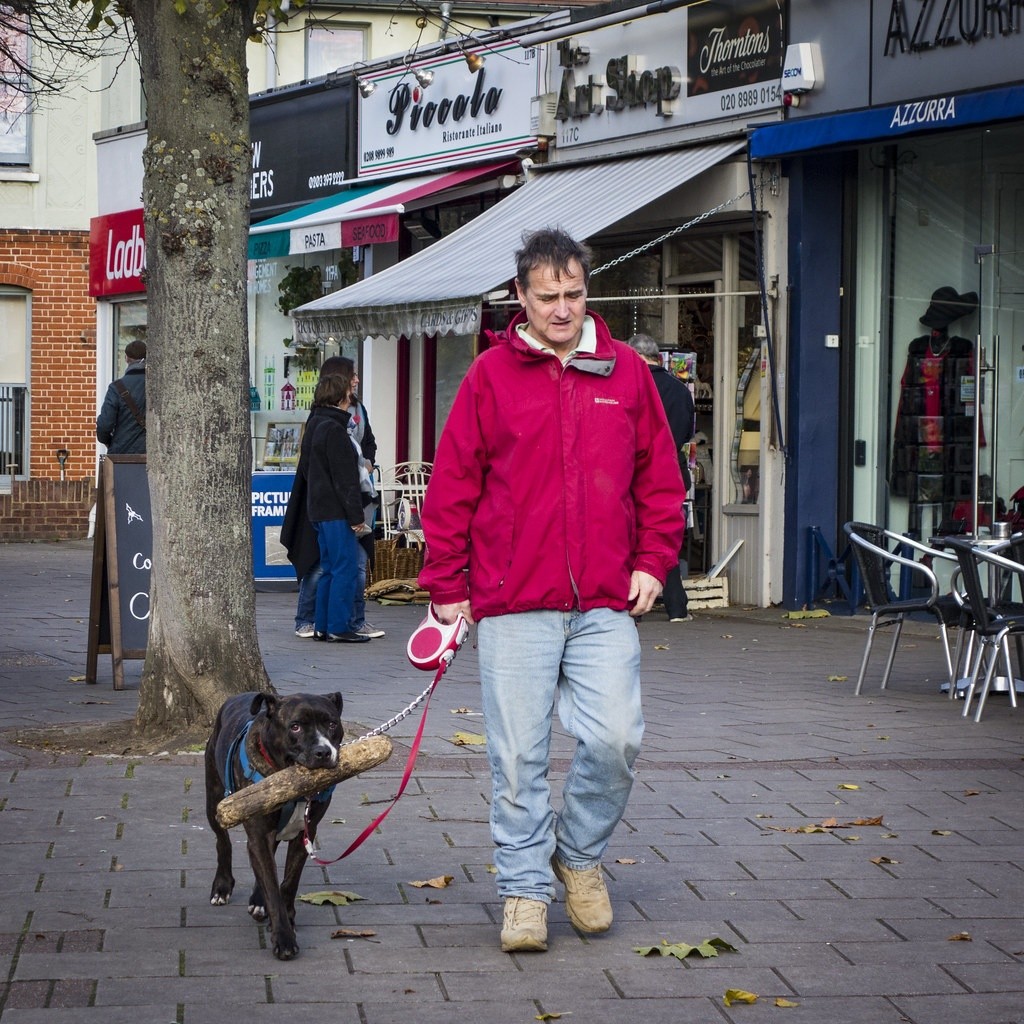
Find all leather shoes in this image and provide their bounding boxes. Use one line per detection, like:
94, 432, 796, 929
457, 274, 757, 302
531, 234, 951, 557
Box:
312, 631, 328, 641
326, 632, 371, 643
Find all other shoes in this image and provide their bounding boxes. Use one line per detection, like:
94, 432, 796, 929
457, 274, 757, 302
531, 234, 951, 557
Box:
670, 613, 693, 622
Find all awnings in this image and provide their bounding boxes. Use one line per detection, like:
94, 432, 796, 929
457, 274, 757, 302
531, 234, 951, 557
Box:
289, 139, 748, 344
247, 159, 521, 259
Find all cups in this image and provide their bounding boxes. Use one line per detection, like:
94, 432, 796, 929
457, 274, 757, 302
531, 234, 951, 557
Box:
991, 521, 1011, 538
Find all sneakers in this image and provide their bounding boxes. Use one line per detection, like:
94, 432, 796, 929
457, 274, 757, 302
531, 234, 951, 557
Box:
551, 848, 613, 933
355, 622, 385, 637
500, 897, 548, 950
295, 624, 316, 637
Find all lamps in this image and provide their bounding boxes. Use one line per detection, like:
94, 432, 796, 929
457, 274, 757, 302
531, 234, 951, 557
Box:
351, 50, 485, 98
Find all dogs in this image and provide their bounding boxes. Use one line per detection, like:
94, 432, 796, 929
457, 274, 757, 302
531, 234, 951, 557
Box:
205, 692, 344, 961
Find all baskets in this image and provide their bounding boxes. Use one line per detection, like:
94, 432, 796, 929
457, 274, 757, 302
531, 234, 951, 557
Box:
365, 530, 425, 588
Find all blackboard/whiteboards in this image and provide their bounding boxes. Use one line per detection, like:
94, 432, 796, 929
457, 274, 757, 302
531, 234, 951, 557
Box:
88, 454, 152, 659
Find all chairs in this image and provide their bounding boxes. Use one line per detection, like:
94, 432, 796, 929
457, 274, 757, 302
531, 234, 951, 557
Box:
844, 521, 1024, 724
380, 462, 433, 545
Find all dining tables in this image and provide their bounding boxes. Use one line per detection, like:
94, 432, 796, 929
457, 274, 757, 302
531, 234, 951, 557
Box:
929, 535, 1024, 696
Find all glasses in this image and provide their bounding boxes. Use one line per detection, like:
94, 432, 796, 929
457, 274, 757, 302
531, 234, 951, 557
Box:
352, 372, 358, 380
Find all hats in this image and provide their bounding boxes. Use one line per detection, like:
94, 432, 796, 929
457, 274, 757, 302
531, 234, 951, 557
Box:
125, 340, 147, 359
919, 285, 978, 327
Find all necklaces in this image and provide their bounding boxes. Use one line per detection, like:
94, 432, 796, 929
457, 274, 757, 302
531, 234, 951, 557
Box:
930, 335, 950, 357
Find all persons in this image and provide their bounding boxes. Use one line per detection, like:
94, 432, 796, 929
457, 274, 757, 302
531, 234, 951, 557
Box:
418, 225, 686, 949
279, 356, 385, 644
623, 330, 693, 624
890, 286, 985, 505
96, 341, 147, 455
739, 346, 761, 468
